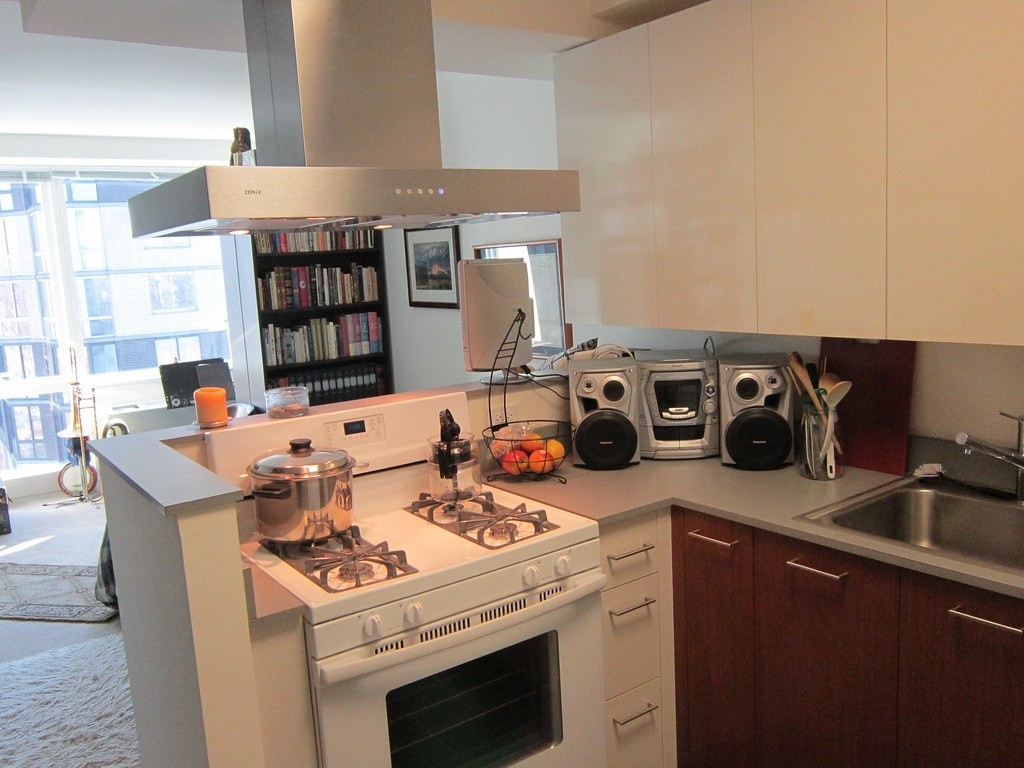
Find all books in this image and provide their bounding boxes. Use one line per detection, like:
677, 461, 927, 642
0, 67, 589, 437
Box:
252, 228, 390, 406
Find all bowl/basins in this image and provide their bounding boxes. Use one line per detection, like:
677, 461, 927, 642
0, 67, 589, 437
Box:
264, 386, 310, 418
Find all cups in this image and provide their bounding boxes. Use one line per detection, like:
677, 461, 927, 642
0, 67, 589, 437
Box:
799, 406, 843, 480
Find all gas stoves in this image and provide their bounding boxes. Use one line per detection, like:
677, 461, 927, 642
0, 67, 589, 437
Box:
203, 388, 600, 660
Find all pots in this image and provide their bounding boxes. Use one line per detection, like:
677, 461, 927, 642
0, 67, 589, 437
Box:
246, 438, 369, 545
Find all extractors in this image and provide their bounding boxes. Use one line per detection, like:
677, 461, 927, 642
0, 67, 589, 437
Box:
127, 0, 581, 239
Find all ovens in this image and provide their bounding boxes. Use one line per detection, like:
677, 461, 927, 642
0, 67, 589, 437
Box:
306, 568, 608, 768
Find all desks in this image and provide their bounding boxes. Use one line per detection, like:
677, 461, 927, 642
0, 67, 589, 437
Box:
108, 394, 265, 436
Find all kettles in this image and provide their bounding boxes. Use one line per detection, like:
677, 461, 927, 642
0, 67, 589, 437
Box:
426, 409, 482, 505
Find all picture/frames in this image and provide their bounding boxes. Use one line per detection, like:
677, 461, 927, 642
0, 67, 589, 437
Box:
470, 238, 572, 362
401, 225, 461, 309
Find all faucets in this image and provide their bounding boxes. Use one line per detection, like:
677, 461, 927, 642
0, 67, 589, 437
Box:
957, 409, 1024, 504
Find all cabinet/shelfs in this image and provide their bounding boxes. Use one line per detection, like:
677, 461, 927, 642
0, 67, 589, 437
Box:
218, 228, 397, 413
554, 0, 1024, 348
589, 506, 1024, 767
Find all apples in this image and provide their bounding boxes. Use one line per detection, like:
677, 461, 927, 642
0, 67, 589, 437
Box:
490, 432, 565, 474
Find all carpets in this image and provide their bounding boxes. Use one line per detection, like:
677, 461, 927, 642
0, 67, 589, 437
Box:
0, 633, 142, 767
0, 562, 120, 623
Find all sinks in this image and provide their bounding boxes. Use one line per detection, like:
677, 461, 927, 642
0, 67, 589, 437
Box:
828, 479, 1024, 575
